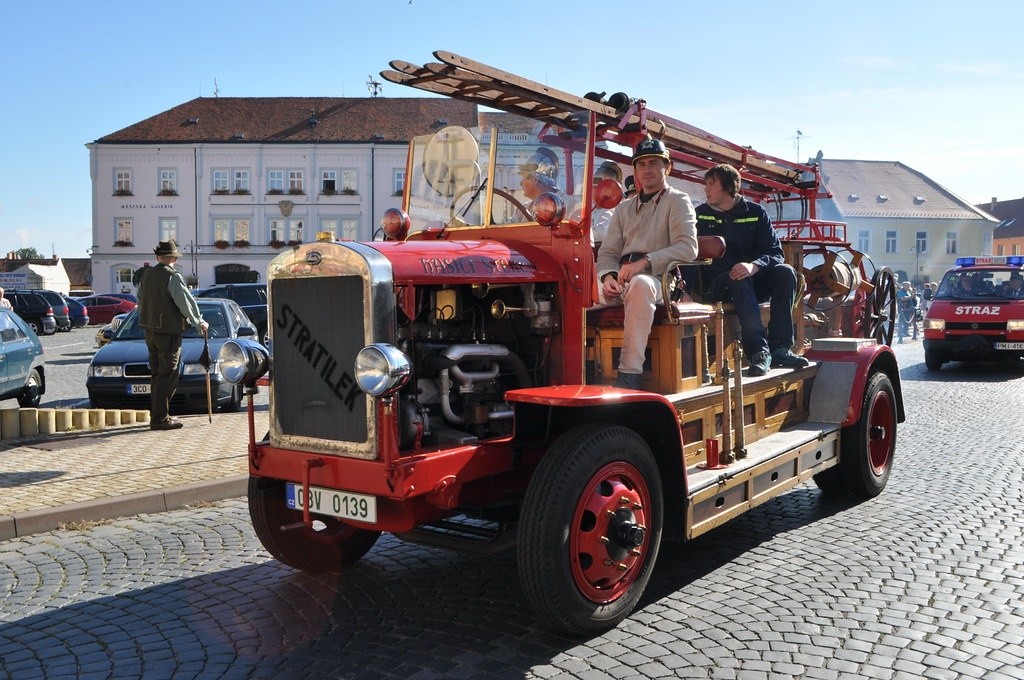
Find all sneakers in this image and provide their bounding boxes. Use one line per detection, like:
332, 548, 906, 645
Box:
747, 351, 772, 375
770, 348, 809, 366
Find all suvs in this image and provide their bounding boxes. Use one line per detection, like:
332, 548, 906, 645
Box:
3, 289, 72, 336
95, 282, 268, 350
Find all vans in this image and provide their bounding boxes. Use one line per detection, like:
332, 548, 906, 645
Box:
923, 255, 1024, 373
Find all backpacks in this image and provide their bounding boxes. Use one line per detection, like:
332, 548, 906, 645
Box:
923, 288, 932, 300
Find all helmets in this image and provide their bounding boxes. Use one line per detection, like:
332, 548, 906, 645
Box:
524, 153, 559, 190
632, 139, 670, 164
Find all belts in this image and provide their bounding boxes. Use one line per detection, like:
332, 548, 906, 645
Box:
619, 254, 642, 262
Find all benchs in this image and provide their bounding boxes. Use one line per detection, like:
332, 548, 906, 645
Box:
584, 236, 727, 396
683, 292, 798, 373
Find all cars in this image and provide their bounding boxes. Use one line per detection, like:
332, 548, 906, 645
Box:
86, 296, 260, 412
57, 293, 139, 327
0, 289, 46, 409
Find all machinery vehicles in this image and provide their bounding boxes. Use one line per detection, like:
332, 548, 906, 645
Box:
216, 49, 906, 637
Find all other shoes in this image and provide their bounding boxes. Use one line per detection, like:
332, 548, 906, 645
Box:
614, 371, 643, 390
901, 331, 910, 337
150, 417, 183, 430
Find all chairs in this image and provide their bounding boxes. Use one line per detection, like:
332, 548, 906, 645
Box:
204, 313, 227, 337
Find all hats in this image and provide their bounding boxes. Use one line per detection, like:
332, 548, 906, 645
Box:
593, 161, 623, 188
152, 239, 183, 257
624, 175, 636, 195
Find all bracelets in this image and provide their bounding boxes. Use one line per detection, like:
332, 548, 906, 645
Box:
643, 255, 651, 272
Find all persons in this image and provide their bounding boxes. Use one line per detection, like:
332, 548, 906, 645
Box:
533, 139, 698, 390
568, 161, 628, 243
679, 163, 809, 377
624, 174, 637, 200
504, 147, 581, 226
137, 238, 209, 431
953, 274, 982, 297
999, 275, 1024, 298
894, 273, 940, 337
